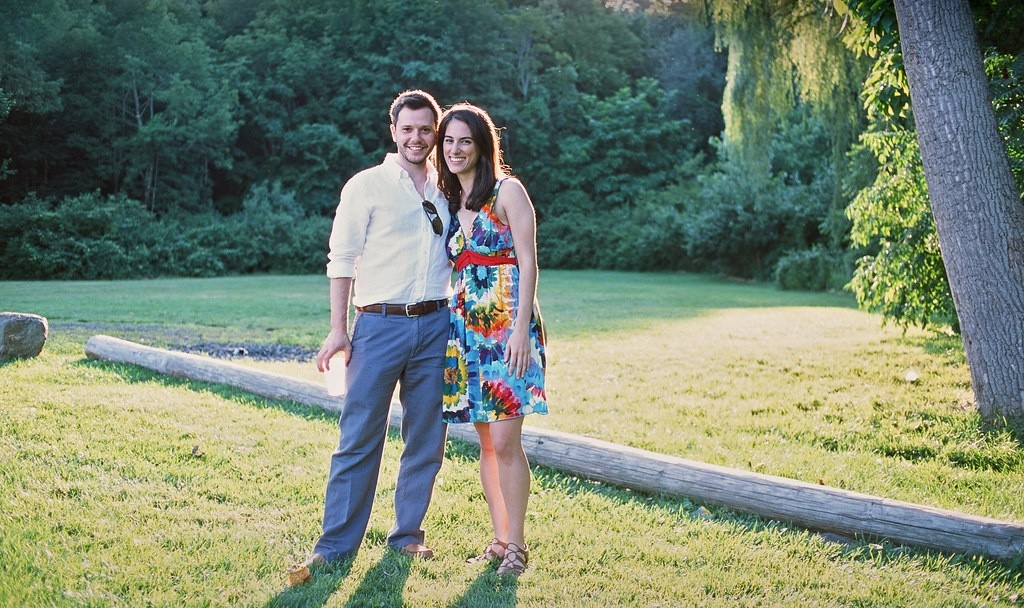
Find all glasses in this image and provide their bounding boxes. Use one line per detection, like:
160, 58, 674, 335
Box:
422, 200, 443, 237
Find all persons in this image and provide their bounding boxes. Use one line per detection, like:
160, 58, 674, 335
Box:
436, 105, 548, 576
290, 88, 454, 574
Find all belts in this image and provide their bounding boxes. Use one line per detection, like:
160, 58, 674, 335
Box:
356, 298, 449, 318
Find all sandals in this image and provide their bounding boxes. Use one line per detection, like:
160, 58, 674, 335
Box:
466, 538, 507, 566
497, 543, 528, 576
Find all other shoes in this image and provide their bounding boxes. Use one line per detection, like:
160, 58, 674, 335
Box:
401, 544, 434, 558
300, 553, 325, 568
